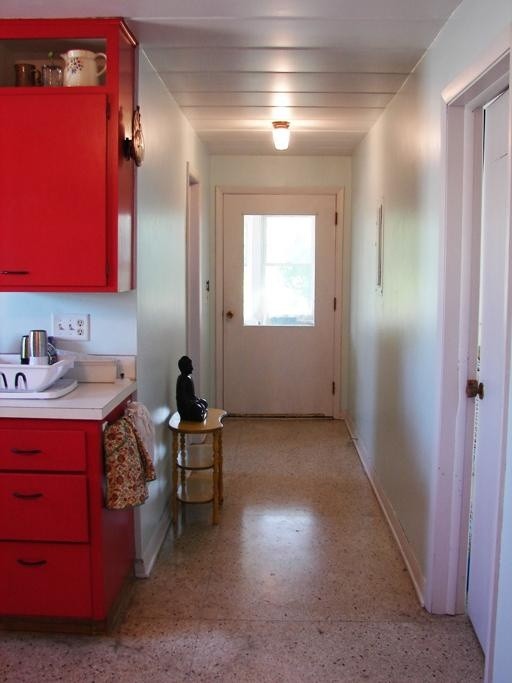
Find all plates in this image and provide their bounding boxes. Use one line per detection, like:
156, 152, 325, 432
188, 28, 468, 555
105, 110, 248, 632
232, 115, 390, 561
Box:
47, 342, 58, 365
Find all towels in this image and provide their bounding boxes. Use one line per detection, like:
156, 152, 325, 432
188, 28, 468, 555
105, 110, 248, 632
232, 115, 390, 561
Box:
104, 419, 149, 512
122, 401, 158, 482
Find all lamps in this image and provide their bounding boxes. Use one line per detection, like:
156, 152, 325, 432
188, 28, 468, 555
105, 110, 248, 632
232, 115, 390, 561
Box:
272, 122, 290, 150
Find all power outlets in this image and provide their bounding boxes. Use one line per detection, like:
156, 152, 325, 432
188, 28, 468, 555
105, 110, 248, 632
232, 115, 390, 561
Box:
103, 422, 108, 431
52, 313, 91, 342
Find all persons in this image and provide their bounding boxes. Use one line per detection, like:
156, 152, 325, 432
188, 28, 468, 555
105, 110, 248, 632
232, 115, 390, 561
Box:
176, 356, 208, 422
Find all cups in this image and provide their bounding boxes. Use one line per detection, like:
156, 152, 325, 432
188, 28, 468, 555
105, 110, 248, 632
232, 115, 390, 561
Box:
59, 48, 107, 86
29, 329, 48, 365
41, 64, 63, 86
20, 335, 30, 365
14, 60, 42, 87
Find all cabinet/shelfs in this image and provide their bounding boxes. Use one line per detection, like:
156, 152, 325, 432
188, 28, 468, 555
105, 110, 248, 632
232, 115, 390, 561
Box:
0, 15, 145, 294
0, 395, 137, 635
168, 408, 226, 524
